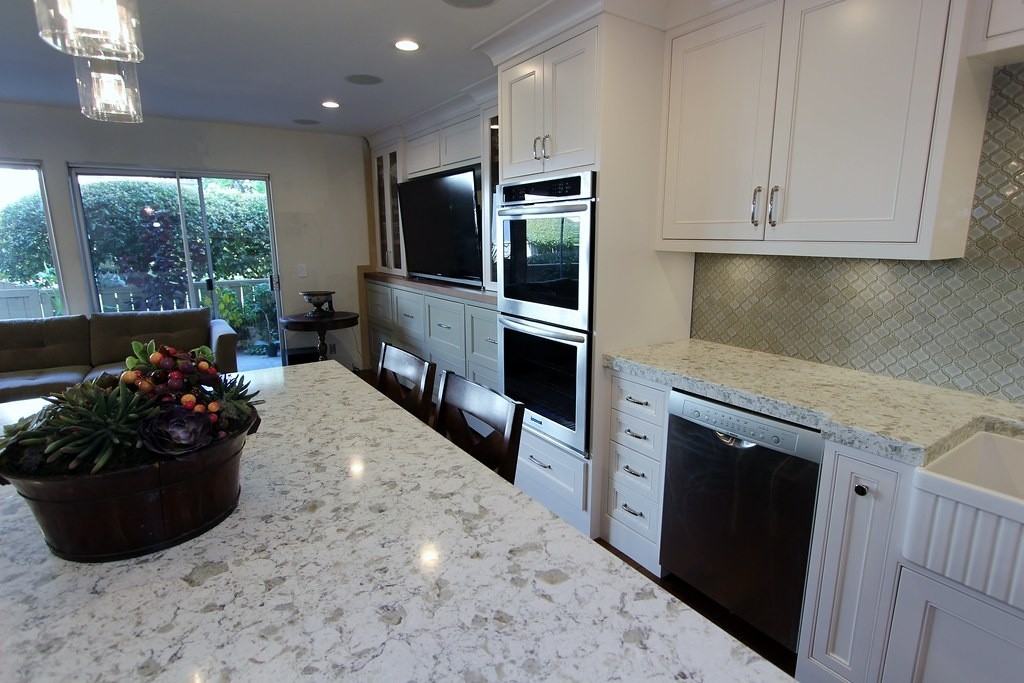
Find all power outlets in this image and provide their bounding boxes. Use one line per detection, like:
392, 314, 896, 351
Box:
330, 343, 336, 355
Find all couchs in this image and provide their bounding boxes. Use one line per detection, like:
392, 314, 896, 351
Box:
0, 306, 239, 404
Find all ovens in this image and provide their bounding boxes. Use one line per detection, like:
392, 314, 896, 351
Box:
496, 170, 596, 462
660, 388, 824, 678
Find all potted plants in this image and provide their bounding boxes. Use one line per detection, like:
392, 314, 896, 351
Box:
242, 307, 259, 337
1, 338, 266, 563
266, 336, 278, 356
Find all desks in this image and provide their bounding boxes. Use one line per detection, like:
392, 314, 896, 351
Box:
194, 279, 269, 337
1, 356, 792, 682
280, 312, 360, 361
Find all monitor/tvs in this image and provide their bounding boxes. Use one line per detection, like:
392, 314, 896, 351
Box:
397, 167, 484, 291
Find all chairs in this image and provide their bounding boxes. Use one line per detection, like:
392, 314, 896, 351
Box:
351, 341, 437, 417
430, 370, 525, 485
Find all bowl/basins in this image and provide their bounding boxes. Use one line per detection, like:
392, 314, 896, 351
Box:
0, 406, 258, 564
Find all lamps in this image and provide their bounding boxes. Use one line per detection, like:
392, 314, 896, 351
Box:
34, 0, 146, 64
74, 56, 143, 124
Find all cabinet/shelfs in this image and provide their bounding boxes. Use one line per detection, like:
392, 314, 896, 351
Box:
425, 297, 467, 402
481, 99, 504, 290
969, 1, 1024, 61
404, 115, 483, 179
498, 15, 609, 178
601, 371, 673, 578
521, 423, 589, 509
393, 289, 426, 387
367, 139, 408, 275
465, 303, 499, 391
879, 560, 1024, 682
650, 0, 991, 261
367, 283, 399, 367
791, 439, 915, 681
